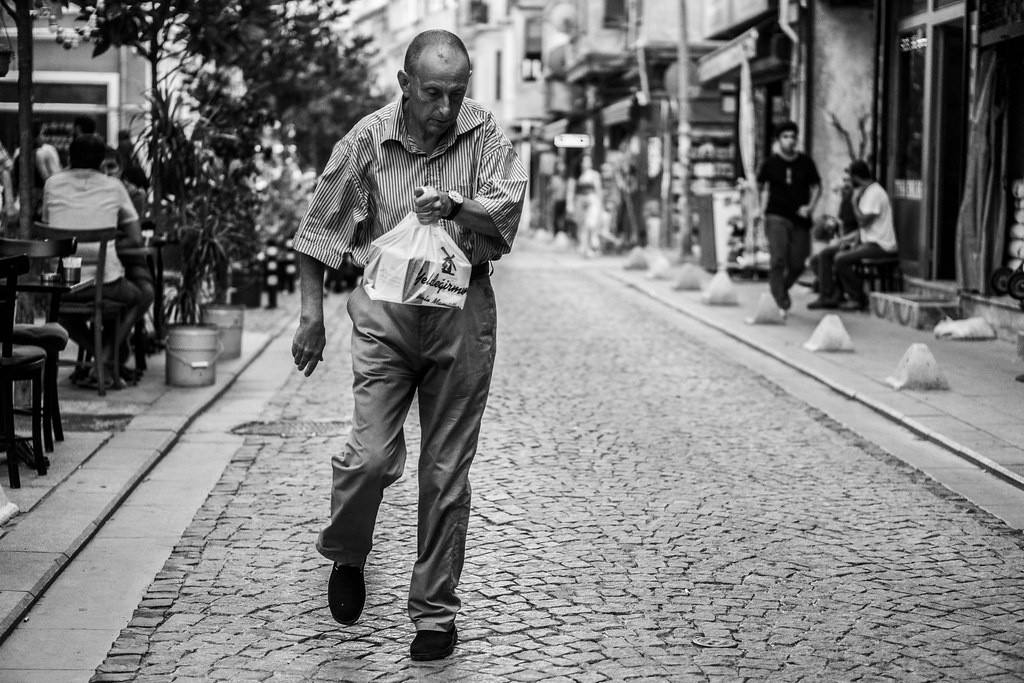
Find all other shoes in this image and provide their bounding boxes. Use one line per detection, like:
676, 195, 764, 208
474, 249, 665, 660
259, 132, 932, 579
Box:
806, 294, 841, 309
777, 289, 791, 318
838, 300, 866, 312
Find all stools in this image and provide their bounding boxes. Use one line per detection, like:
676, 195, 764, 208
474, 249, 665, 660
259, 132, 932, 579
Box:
855, 259, 907, 310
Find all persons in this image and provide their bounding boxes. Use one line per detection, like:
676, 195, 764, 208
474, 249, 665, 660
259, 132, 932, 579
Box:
756, 120, 824, 316
806, 159, 898, 310
545, 152, 628, 254
0, 115, 155, 386
291, 29, 523, 662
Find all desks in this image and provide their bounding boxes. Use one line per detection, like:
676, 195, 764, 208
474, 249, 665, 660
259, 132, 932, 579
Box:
0, 271, 97, 417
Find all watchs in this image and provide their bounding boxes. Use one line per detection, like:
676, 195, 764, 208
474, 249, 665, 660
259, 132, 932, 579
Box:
442, 188, 465, 222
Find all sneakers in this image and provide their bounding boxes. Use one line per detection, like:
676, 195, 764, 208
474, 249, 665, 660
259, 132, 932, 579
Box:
410, 622, 458, 661
328, 557, 366, 625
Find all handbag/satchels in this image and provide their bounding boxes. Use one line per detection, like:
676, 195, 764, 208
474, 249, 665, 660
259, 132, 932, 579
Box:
361, 188, 472, 310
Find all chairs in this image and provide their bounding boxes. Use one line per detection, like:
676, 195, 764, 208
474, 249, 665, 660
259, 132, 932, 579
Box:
0, 218, 169, 492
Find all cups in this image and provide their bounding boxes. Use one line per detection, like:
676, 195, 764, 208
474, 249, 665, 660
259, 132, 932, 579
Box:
62, 257, 82, 282
142, 229, 154, 247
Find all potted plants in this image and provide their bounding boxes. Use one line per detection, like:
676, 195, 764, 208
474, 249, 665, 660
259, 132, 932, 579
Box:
128, 81, 262, 392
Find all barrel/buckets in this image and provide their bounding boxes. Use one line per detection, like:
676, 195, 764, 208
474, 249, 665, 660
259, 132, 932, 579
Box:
202, 304, 245, 359
165, 324, 223, 387
